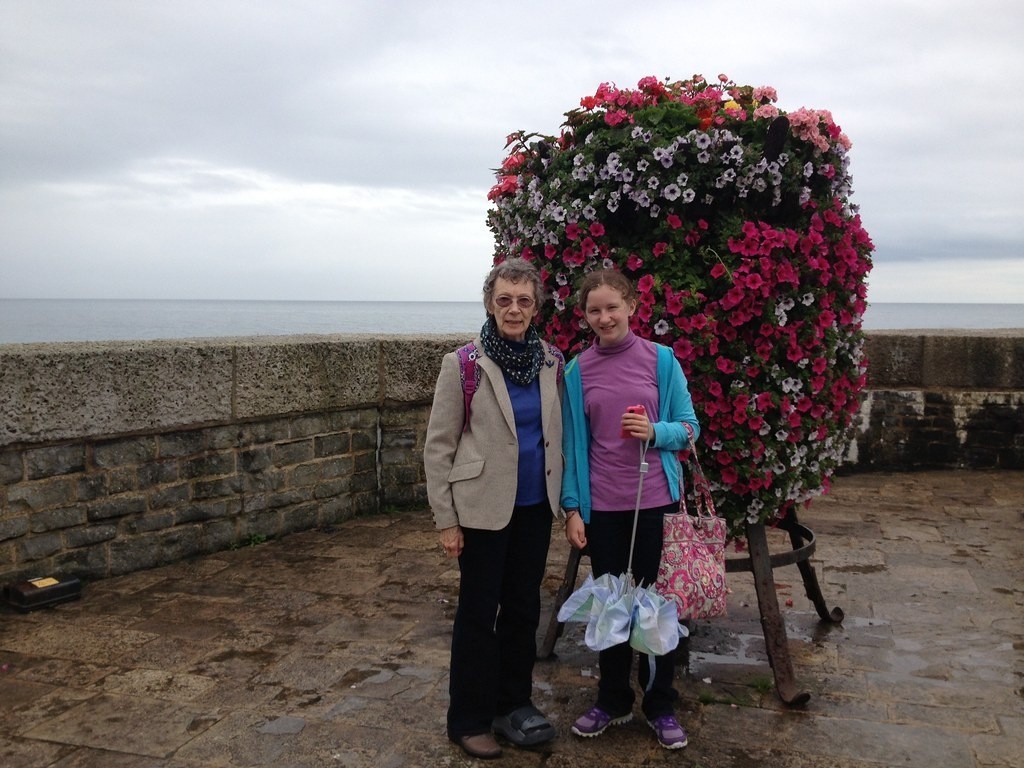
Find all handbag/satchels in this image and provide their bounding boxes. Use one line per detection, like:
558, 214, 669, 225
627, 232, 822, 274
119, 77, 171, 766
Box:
656, 423, 727, 620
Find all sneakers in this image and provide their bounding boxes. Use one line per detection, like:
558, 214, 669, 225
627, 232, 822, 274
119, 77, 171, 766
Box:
647, 715, 688, 749
571, 706, 632, 737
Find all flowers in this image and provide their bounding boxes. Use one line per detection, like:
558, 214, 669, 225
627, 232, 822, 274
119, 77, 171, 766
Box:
485, 72, 873, 550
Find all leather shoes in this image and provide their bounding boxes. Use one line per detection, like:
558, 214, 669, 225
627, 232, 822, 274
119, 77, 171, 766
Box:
448, 729, 502, 760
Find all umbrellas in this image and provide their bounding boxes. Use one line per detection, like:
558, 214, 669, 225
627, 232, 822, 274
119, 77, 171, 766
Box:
557, 462, 689, 689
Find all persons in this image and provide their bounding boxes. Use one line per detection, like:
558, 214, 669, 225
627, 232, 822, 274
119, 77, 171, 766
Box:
422, 257, 564, 756
559, 269, 700, 748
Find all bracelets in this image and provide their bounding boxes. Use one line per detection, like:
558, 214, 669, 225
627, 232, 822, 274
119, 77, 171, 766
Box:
564, 512, 575, 523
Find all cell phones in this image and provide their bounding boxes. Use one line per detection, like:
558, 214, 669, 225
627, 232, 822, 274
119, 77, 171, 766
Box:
619, 406, 646, 439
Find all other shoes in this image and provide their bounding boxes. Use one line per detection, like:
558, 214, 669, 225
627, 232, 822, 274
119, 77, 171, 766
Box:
490, 707, 555, 747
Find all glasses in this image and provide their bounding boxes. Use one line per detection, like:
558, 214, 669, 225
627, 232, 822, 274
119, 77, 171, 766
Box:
491, 296, 536, 309
